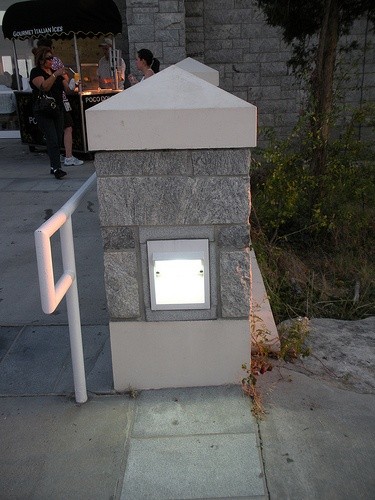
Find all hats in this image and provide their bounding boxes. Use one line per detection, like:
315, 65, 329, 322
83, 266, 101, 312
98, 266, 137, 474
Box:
98, 38, 112, 46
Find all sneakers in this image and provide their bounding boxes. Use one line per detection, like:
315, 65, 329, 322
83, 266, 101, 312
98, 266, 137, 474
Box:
60, 155, 66, 162
65, 156, 83, 165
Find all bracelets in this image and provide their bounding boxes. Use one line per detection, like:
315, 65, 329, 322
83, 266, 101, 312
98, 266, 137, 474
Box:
52, 73, 56, 78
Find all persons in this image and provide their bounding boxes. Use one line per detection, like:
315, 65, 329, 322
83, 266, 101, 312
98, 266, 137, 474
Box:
96, 38, 126, 90
29, 46, 68, 178
128, 48, 160, 85
37, 38, 84, 166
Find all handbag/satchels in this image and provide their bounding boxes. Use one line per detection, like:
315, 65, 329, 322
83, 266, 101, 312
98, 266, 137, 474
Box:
39, 94, 59, 117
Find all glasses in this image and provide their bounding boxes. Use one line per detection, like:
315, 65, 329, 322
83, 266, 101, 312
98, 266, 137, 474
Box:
46, 56, 53, 61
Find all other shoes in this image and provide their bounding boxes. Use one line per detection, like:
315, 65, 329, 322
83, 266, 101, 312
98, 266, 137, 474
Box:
54, 169, 64, 178
51, 167, 65, 175
